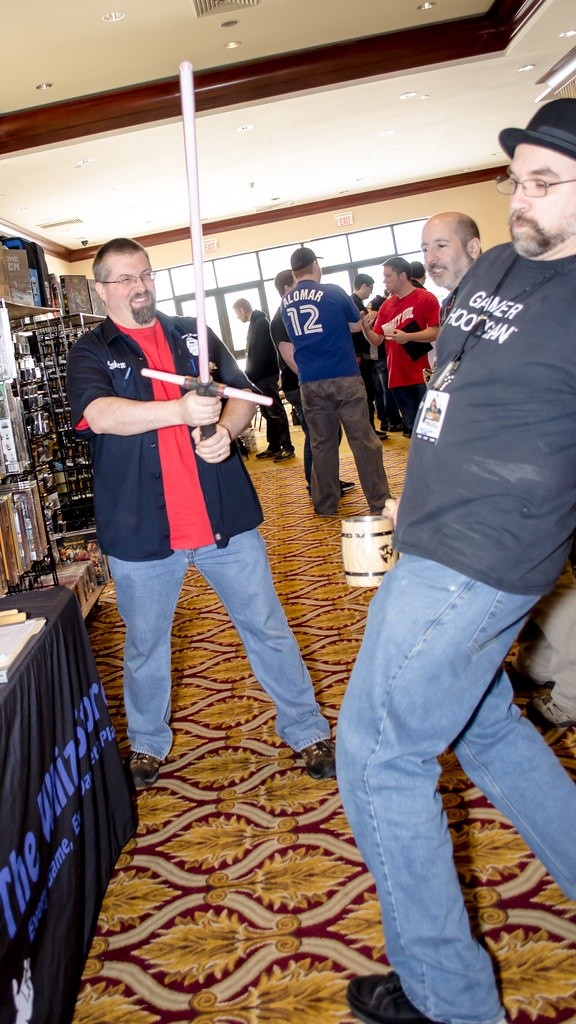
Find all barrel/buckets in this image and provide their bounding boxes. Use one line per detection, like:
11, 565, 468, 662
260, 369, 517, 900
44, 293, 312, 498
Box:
341, 514, 400, 589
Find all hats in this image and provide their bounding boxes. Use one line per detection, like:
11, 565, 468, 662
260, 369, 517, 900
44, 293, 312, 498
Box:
498, 98, 576, 161
291, 248, 323, 271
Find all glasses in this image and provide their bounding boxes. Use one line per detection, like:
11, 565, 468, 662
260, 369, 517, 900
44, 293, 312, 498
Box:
495, 175, 576, 197
366, 284, 373, 290
99, 271, 156, 286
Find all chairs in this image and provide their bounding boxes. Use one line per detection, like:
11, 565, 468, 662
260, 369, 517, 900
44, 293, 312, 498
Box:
254, 391, 286, 431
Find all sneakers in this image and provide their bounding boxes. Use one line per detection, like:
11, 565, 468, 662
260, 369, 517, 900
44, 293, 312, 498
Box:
340, 481, 355, 490
346, 969, 438, 1024
273, 448, 295, 461
256, 445, 280, 459
300, 739, 336, 779
123, 751, 161, 787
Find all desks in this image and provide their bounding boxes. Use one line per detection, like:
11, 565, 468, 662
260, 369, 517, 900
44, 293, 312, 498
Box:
0, 583, 141, 1024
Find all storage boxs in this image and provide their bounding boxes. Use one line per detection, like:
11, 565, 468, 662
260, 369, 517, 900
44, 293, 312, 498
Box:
31, 242, 53, 307
60, 273, 91, 316
29, 268, 42, 307
87, 277, 108, 317
0, 246, 34, 303
2, 237, 36, 269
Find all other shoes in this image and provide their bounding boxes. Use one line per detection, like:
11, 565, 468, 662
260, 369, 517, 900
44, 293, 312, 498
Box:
402, 433, 410, 438
375, 431, 388, 439
388, 422, 403, 432
380, 425, 387, 430
505, 663, 556, 691
526, 695, 576, 730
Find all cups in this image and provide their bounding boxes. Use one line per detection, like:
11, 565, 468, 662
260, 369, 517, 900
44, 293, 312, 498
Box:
382, 323, 394, 339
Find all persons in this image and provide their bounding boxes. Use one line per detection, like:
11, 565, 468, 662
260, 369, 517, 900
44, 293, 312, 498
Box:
425, 400, 441, 421
345, 96, 576, 1024
368, 289, 404, 431
269, 270, 355, 499
66, 237, 335, 788
360, 257, 442, 432
281, 247, 391, 517
423, 210, 576, 725
233, 298, 295, 462
410, 260, 427, 290
351, 275, 388, 441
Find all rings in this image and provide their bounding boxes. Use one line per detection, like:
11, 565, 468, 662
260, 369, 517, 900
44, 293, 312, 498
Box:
218, 452, 221, 456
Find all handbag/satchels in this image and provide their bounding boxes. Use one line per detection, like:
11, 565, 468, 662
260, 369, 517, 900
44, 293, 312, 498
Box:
290, 408, 300, 426
